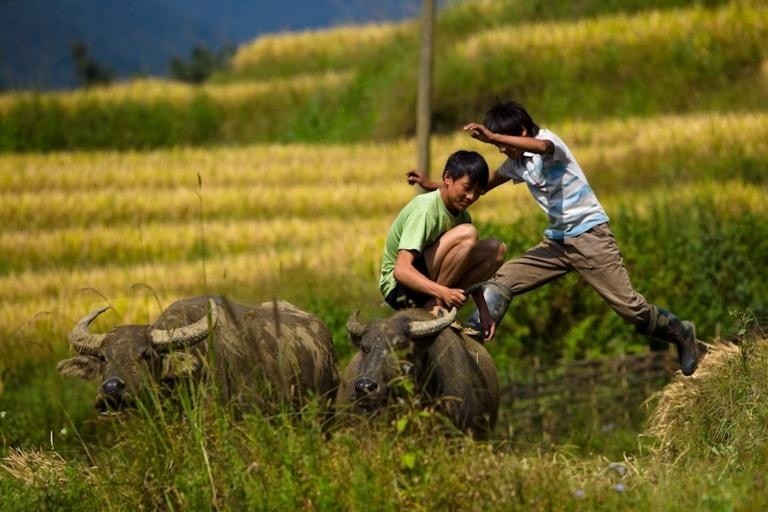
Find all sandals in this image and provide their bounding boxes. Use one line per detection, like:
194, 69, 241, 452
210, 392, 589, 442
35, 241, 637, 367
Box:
427, 305, 464, 332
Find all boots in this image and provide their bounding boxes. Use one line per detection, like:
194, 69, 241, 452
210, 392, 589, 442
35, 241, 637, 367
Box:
451, 281, 514, 343
639, 302, 701, 378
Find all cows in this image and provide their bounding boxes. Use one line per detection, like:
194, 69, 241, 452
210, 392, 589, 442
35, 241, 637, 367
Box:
334, 307, 501, 462
54, 294, 341, 443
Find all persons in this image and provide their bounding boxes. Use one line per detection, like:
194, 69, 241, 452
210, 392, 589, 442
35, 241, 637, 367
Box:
381, 150, 508, 343
407, 101, 699, 376
